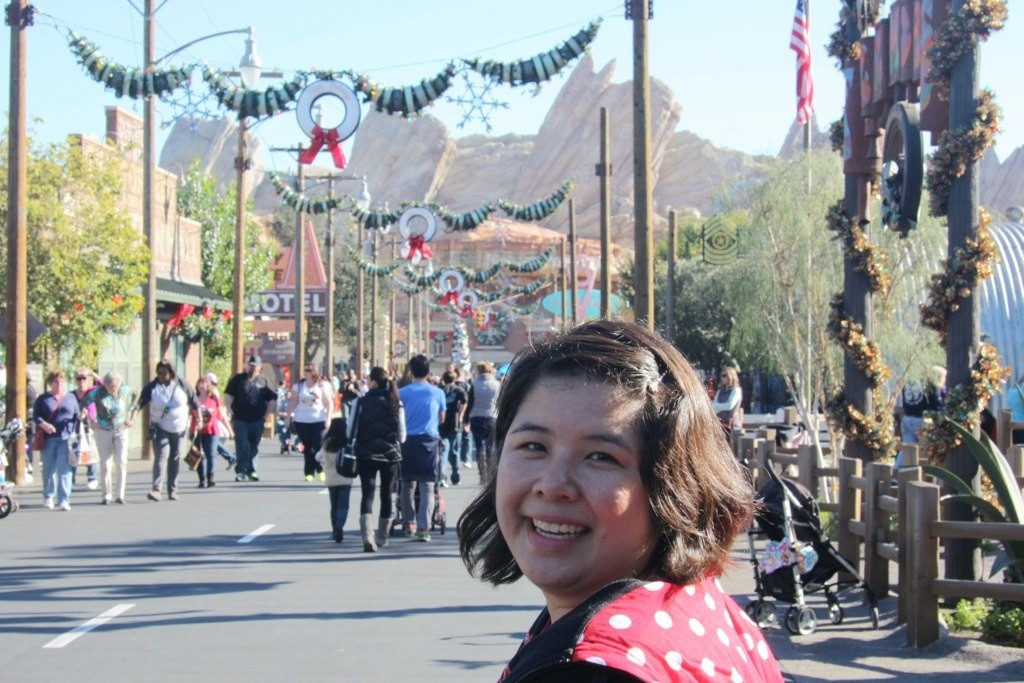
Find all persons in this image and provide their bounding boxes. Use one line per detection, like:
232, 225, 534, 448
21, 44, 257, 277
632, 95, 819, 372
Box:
452, 314, 787, 681
27, 352, 278, 512
711, 364, 742, 442
281, 355, 502, 554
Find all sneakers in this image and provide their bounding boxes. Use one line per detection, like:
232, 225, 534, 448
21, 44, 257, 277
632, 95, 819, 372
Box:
404, 524, 418, 536
417, 529, 430, 542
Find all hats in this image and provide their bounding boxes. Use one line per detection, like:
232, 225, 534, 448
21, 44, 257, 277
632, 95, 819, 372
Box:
207, 373, 217, 384
248, 356, 261, 366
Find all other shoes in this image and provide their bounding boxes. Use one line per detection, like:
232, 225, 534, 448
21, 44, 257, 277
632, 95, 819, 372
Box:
333, 529, 344, 542
305, 474, 314, 482
43, 475, 215, 510
226, 460, 236, 470
249, 472, 259, 481
318, 472, 325, 481
465, 462, 471, 468
235, 473, 244, 481
440, 481, 448, 487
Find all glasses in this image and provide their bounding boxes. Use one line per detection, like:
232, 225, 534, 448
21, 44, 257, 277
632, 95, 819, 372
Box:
77, 376, 88, 381
304, 369, 312, 372
721, 374, 727, 377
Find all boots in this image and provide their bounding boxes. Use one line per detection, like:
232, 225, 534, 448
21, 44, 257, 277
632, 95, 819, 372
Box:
360, 513, 378, 551
375, 516, 393, 548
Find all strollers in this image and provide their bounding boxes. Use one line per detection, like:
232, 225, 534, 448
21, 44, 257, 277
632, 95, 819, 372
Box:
388, 440, 446, 536
736, 456, 881, 637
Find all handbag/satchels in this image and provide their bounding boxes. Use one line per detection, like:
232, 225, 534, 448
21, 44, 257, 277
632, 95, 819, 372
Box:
32, 430, 45, 450
146, 428, 157, 441
184, 428, 204, 472
68, 414, 101, 467
335, 446, 358, 478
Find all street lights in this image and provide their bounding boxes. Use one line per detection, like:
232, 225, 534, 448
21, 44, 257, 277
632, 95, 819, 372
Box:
366, 227, 435, 382
135, 1, 266, 464
355, 198, 393, 382
292, 170, 373, 384
